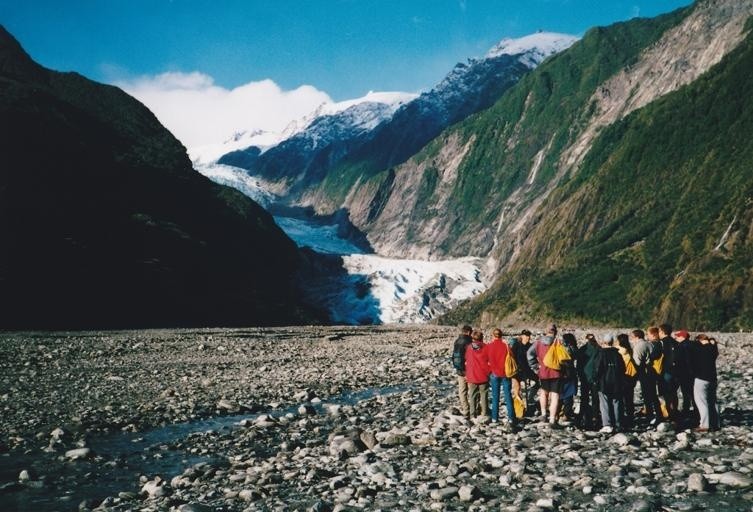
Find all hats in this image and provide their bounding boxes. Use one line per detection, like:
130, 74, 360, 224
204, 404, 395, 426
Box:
603, 334, 613, 344
522, 328, 534, 337
675, 332, 690, 339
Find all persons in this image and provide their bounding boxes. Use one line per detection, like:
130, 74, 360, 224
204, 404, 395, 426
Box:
450, 322, 719, 434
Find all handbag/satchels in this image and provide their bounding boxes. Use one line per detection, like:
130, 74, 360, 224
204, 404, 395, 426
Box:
658, 397, 668, 418
513, 396, 526, 418
652, 354, 664, 374
543, 344, 571, 370
504, 353, 517, 377
622, 354, 636, 376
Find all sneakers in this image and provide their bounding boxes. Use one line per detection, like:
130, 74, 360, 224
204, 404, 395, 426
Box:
538, 415, 560, 428
599, 426, 613, 433
693, 427, 708, 431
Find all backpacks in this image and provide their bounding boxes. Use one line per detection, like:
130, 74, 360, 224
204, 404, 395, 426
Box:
453, 344, 465, 371
